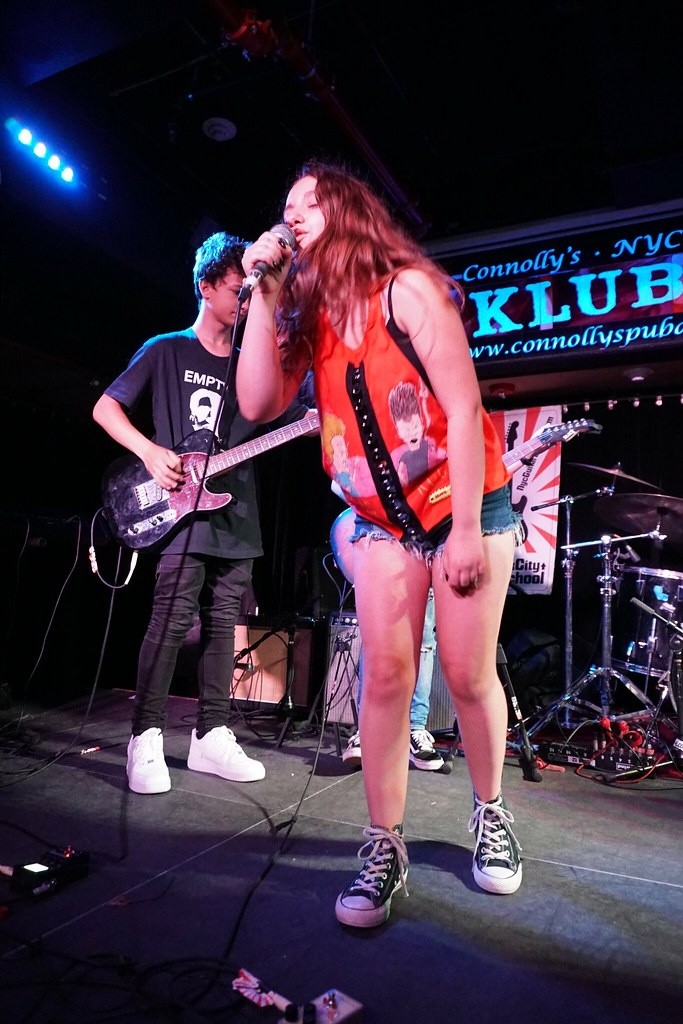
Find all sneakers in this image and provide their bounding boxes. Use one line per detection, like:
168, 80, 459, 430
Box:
342, 730, 362, 768
335, 821, 411, 927
126, 727, 171, 794
187, 725, 266, 782
467, 786, 522, 894
410, 729, 444, 770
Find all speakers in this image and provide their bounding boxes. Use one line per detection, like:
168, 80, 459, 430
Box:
229, 614, 329, 721
322, 610, 460, 740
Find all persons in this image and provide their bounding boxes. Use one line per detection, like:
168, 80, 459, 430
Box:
236, 161, 521, 929
90, 232, 322, 793
330, 422, 555, 771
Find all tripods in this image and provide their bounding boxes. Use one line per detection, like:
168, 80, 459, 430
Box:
234, 592, 324, 749
506, 461, 678, 755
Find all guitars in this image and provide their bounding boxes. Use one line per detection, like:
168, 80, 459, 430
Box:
103, 415, 320, 548
330, 419, 604, 585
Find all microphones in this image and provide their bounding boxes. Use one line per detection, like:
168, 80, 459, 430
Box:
612, 534, 641, 562
235, 662, 254, 672
238, 223, 297, 302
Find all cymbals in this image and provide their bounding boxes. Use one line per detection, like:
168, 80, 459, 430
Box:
564, 461, 663, 491
593, 491, 683, 543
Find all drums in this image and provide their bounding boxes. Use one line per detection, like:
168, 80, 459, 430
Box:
611, 567, 682, 678
